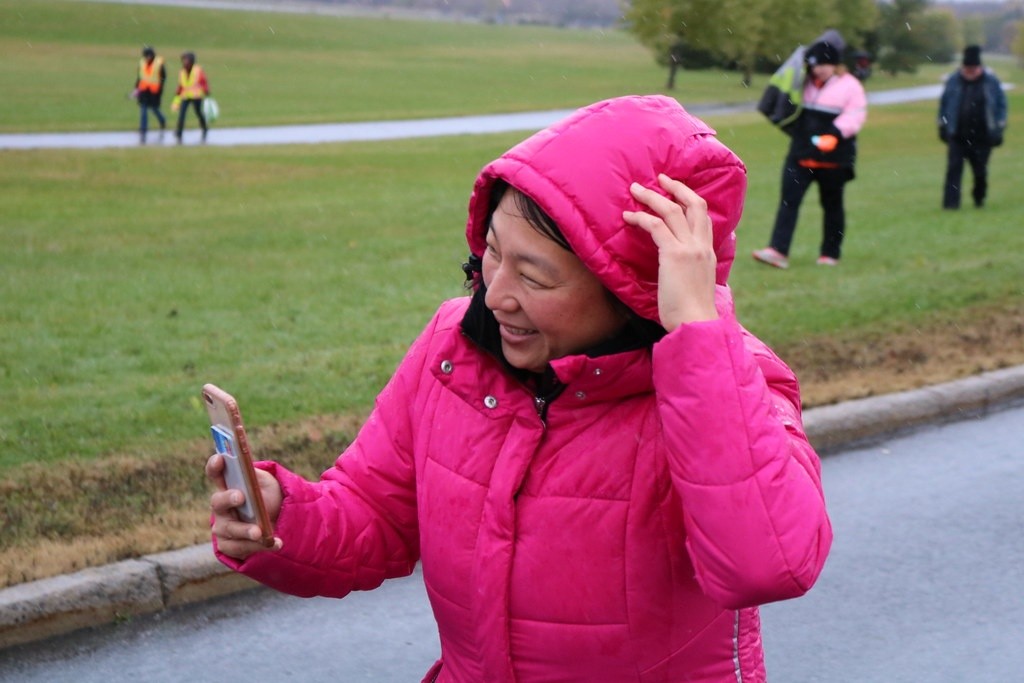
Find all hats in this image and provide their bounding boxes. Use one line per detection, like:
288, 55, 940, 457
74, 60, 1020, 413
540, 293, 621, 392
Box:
964, 46, 982, 65
805, 42, 840, 65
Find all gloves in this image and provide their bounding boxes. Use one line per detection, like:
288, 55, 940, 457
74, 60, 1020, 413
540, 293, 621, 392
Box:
816, 135, 839, 152
939, 126, 951, 144
991, 134, 1003, 146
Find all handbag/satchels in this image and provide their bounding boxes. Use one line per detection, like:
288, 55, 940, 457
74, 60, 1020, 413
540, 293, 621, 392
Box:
203, 96, 219, 121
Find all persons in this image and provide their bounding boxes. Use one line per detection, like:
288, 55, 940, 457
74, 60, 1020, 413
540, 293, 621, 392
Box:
132, 47, 210, 138
937, 43, 1008, 210
752, 41, 869, 270
205, 94, 834, 682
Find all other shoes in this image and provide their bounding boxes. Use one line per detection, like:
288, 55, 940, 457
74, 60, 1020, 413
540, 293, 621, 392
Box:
944, 198, 960, 208
172, 131, 182, 137
973, 191, 982, 208
203, 128, 208, 137
139, 127, 146, 133
160, 120, 165, 128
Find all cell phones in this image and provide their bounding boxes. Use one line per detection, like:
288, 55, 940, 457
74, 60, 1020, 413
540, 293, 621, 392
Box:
200, 382, 275, 548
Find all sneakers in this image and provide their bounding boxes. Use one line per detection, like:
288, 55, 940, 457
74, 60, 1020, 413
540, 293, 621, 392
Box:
816, 256, 839, 264
753, 247, 786, 268
